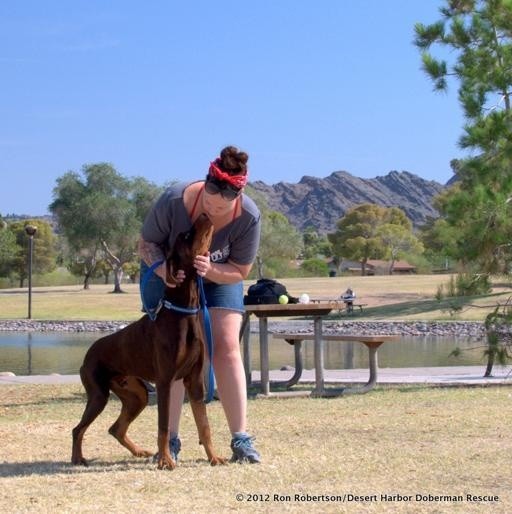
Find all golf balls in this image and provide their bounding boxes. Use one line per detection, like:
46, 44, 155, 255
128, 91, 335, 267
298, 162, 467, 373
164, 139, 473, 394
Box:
299, 294, 309, 305
278, 295, 289, 304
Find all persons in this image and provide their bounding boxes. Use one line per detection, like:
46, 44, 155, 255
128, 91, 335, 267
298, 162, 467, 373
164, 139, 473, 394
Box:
138, 146, 265, 462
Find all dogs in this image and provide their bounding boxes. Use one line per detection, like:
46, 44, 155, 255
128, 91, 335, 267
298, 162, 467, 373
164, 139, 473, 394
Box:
71, 212, 230, 471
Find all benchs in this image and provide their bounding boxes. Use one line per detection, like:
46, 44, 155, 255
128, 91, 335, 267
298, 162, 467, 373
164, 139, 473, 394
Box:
272, 333, 401, 343
353, 304, 368, 312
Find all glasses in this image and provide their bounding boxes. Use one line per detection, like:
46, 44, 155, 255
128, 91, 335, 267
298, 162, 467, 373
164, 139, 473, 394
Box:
204, 178, 243, 202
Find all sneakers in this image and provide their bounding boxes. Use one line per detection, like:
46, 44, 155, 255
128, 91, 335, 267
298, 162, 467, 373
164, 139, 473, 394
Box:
151, 429, 183, 463
229, 431, 262, 465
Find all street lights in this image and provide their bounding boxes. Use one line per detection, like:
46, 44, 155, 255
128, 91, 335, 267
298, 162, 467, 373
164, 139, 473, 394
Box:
25, 225, 37, 318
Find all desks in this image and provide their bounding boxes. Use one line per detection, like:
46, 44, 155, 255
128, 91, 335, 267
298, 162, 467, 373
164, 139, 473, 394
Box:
243, 303, 383, 399
311, 299, 355, 315
